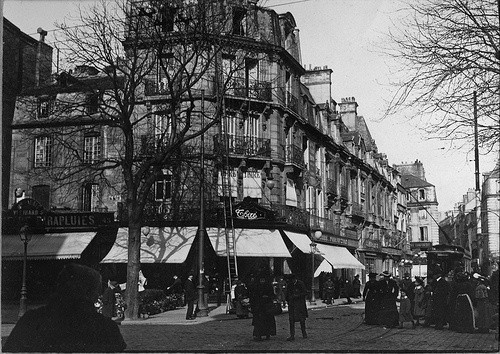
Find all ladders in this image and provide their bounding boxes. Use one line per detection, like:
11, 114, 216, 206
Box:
221, 116, 239, 291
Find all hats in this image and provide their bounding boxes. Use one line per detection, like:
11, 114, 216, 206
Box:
369, 273, 378, 276
56, 263, 101, 302
379, 271, 391, 277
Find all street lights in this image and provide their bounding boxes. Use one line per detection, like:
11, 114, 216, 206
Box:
17, 222, 33, 320
308, 207, 322, 306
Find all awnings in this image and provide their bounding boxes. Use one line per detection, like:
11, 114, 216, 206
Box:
207, 226, 292, 257
284, 228, 319, 254
100, 226, 199, 263
316, 241, 366, 270
1, 230, 98, 261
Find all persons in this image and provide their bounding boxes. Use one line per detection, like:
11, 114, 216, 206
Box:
100, 265, 500, 333
251, 272, 276, 340
1, 264, 127, 352
285, 273, 309, 340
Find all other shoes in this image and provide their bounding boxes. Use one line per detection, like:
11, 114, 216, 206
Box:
410, 326, 415, 330
266, 336, 270, 340
253, 337, 261, 342
303, 333, 307, 339
286, 336, 294, 341
398, 326, 403, 329
